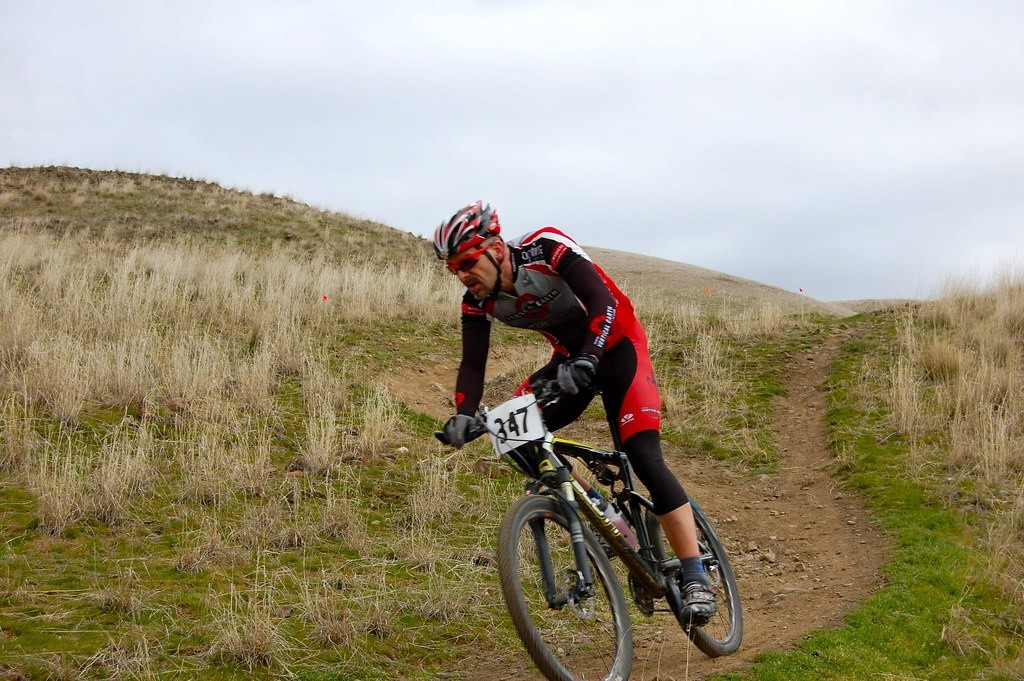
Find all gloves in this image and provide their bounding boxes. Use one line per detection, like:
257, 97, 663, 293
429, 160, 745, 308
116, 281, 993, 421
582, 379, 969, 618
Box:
442, 414, 481, 450
556, 353, 600, 395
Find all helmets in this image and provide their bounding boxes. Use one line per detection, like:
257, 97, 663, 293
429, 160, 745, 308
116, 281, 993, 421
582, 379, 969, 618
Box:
432, 198, 501, 260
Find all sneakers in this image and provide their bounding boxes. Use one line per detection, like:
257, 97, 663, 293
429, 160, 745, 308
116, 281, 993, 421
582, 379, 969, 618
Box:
680, 572, 718, 625
589, 509, 632, 557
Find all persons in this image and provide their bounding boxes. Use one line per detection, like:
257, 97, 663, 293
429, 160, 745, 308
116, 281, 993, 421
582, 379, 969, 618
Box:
432, 201, 717, 617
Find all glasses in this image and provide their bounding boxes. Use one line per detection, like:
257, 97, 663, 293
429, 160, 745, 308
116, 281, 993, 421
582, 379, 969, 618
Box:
446, 240, 494, 275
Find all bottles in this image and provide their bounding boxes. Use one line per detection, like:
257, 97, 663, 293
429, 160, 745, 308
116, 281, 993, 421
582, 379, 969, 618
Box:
591, 497, 639, 549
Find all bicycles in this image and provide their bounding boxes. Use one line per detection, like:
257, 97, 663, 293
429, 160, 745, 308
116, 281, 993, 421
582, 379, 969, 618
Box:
435, 358, 743, 681
